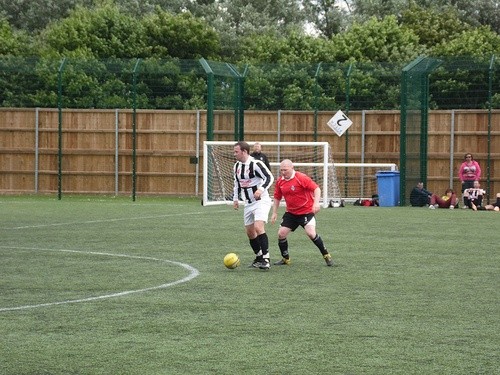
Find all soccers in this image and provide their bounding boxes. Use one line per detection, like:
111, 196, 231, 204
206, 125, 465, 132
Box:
441, 196, 449, 201
222, 252, 241, 270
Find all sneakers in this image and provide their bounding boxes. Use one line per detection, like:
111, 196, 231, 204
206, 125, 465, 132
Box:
273, 259, 291, 265
323, 254, 334, 266
250, 258, 262, 268
259, 259, 271, 270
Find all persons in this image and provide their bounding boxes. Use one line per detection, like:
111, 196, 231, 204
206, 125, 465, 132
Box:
232, 142, 274, 269
462, 181, 486, 208
249, 143, 272, 171
271, 159, 333, 267
458, 153, 481, 193
471, 193, 500, 211
429, 189, 459, 209
410, 181, 432, 206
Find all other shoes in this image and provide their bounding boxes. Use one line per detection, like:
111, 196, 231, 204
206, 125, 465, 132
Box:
450, 206, 454, 210
429, 205, 434, 209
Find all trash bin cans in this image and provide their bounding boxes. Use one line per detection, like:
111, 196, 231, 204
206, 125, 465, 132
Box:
375, 171, 400, 207
